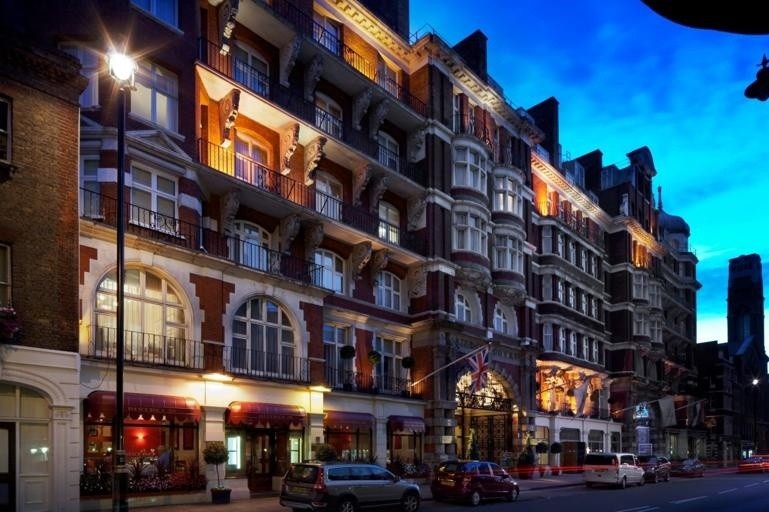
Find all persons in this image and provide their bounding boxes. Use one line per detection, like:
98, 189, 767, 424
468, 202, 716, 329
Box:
158, 445, 169, 469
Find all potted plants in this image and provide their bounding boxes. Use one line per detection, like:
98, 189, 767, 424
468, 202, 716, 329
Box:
340, 345, 356, 392
203, 443, 232, 504
536, 441, 563, 477
400, 357, 415, 398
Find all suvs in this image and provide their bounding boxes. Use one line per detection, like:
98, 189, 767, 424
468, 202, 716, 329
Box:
278, 461, 422, 512
582, 452, 645, 490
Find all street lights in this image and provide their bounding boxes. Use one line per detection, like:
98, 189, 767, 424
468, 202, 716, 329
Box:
751, 376, 760, 453
104, 34, 141, 511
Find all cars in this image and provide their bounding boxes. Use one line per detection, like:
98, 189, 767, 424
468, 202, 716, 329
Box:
637, 455, 672, 484
430, 459, 521, 507
677, 459, 706, 477
736, 456, 769, 473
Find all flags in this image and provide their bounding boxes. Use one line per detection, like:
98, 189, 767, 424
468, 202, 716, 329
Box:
466, 344, 490, 394
657, 397, 678, 427
572, 378, 589, 417
690, 400, 704, 427
